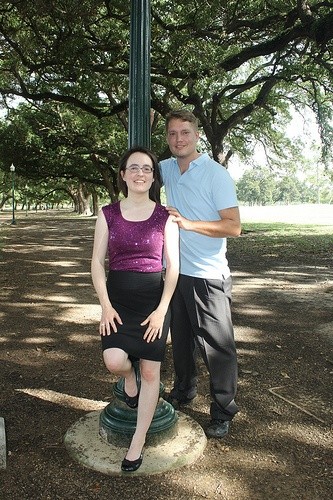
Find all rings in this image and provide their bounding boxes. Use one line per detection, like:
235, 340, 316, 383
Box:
102, 324, 105, 326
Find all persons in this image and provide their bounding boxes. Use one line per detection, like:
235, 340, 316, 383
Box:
91, 146, 179, 472
157, 110, 242, 439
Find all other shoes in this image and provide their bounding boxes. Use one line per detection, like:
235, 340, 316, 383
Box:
205, 418, 229, 438
167, 391, 196, 410
121, 436, 147, 471
123, 366, 139, 409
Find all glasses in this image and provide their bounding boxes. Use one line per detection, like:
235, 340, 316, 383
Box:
124, 166, 154, 173
